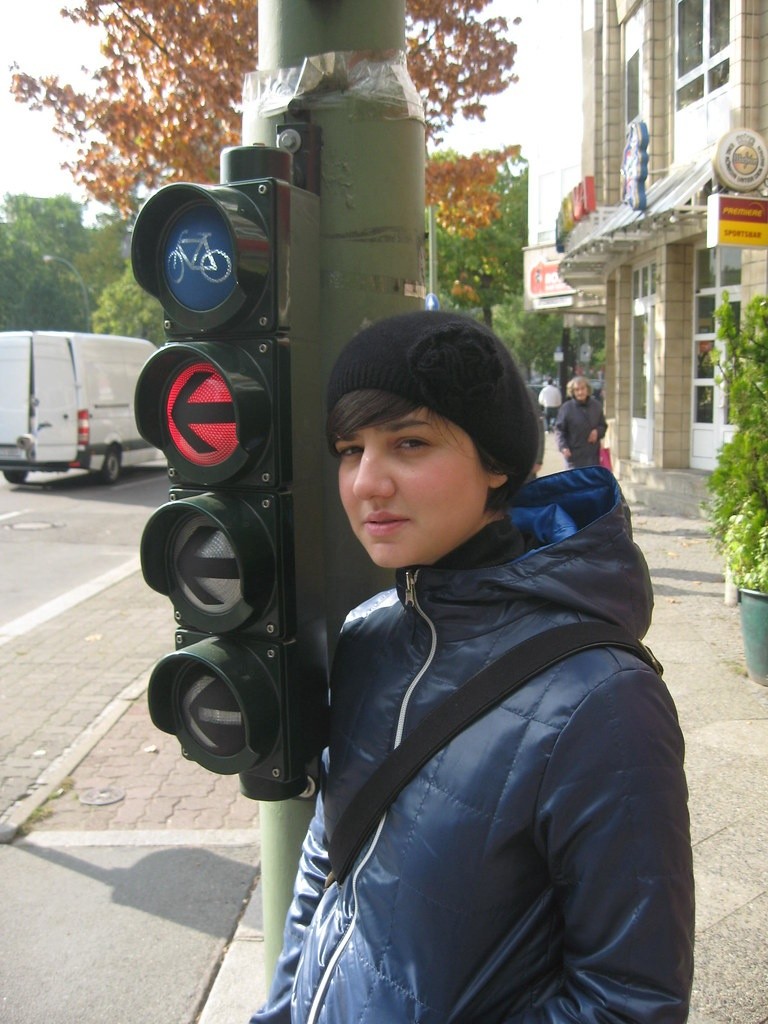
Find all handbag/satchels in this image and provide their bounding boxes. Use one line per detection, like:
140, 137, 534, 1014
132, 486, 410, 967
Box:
599, 428, 613, 471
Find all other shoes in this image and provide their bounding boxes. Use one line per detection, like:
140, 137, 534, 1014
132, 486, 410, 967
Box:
545, 431, 549, 436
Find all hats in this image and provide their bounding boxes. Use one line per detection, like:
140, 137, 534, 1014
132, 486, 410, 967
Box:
324, 309, 539, 472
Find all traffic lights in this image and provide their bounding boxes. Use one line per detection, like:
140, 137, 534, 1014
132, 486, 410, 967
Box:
131, 143, 324, 802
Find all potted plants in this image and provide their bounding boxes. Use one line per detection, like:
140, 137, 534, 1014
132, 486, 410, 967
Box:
697, 289, 768, 687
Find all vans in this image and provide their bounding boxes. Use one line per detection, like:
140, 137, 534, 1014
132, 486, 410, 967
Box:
0, 331, 161, 484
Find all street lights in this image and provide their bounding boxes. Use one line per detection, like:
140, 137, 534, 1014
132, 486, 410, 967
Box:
44, 254, 93, 333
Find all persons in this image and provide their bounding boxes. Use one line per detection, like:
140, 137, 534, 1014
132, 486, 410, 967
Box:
538, 379, 562, 433
554, 375, 608, 469
246, 312, 697, 1023
